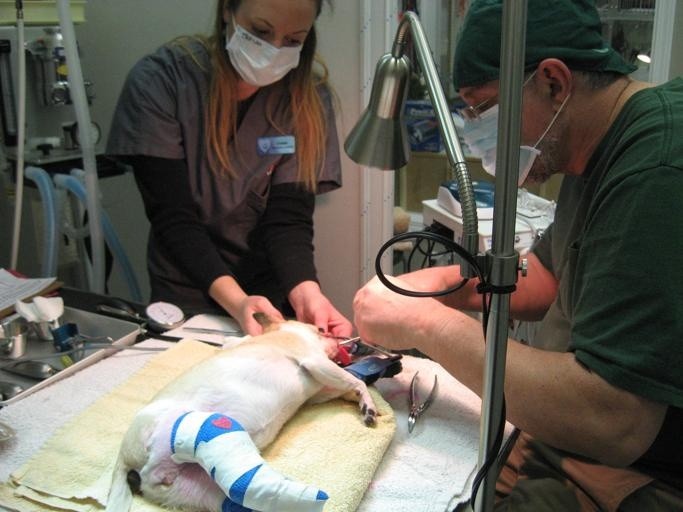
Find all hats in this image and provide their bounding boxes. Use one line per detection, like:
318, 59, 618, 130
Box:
450, 0, 642, 93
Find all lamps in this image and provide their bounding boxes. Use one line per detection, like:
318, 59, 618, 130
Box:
344, 0, 528, 512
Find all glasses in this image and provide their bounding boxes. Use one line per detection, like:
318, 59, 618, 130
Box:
456, 83, 502, 124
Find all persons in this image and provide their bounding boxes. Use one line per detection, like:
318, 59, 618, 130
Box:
351, 0, 682, 511
103, 2, 356, 346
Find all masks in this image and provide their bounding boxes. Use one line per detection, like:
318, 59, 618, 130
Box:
221, 9, 306, 87
459, 68, 564, 188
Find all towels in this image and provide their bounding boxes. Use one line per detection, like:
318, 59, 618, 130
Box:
2, 340, 397, 512
